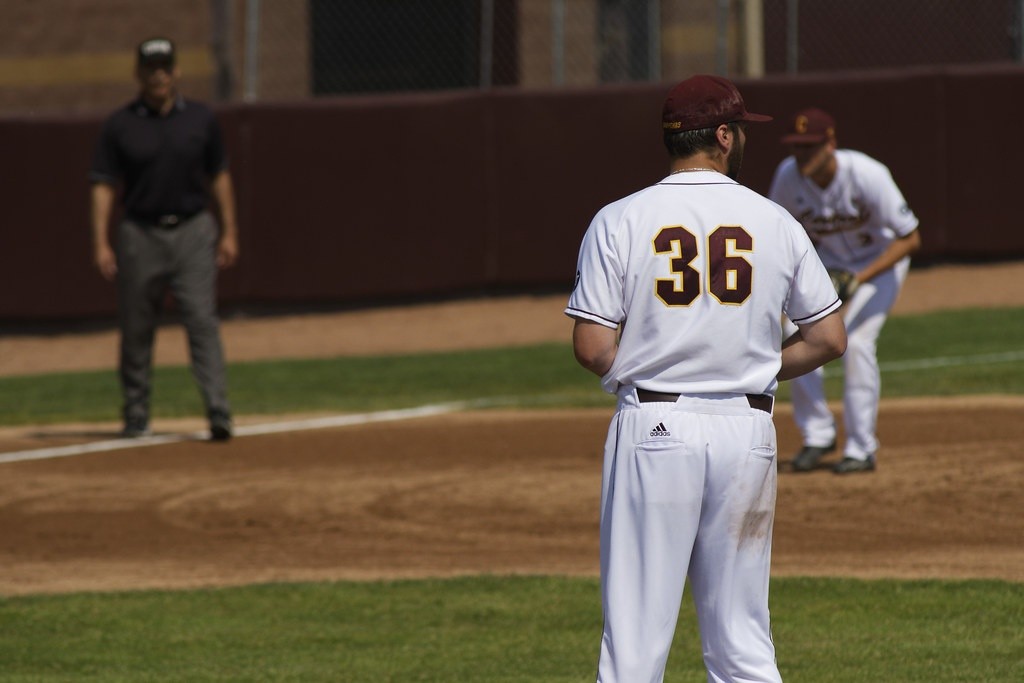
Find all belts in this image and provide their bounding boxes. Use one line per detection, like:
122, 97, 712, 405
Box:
636, 388, 772, 413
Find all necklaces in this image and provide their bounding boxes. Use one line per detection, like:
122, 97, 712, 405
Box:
673, 168, 717, 174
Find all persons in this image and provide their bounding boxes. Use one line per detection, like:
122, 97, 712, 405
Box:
769, 107, 921, 475
91, 37, 237, 440
564, 74, 847, 683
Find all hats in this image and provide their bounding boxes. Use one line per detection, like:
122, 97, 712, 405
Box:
662, 74, 774, 134
782, 107, 836, 142
136, 38, 175, 69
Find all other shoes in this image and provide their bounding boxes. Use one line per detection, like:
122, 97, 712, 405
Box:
210, 426, 229, 441
834, 452, 875, 472
789, 438, 836, 471
